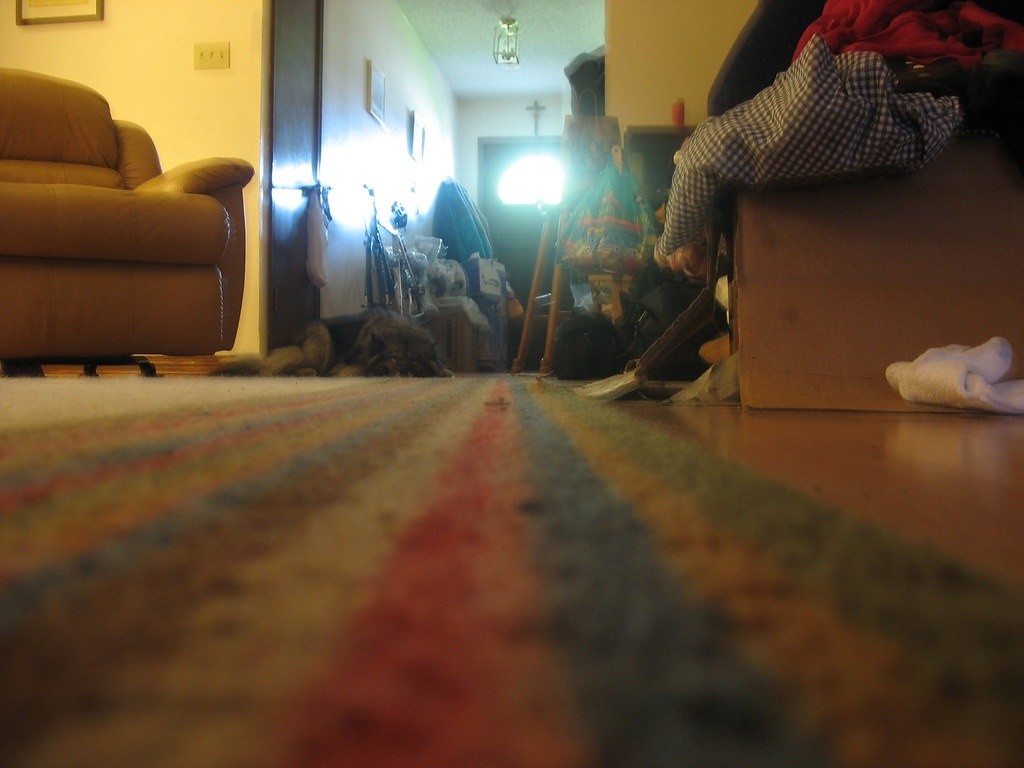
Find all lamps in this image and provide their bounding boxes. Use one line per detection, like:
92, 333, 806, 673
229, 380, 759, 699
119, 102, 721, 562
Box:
494, 17, 520, 65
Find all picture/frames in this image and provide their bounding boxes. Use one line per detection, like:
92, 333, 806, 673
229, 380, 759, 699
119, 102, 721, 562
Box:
409, 109, 427, 165
16, 0, 105, 25
367, 59, 386, 124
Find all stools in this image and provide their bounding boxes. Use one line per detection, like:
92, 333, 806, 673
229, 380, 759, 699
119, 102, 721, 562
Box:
511, 195, 602, 375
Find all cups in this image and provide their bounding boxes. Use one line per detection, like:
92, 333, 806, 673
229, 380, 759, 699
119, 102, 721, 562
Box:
672, 98, 684, 125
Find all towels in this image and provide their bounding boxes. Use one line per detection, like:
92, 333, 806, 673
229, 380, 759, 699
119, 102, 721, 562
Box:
883, 336, 1024, 417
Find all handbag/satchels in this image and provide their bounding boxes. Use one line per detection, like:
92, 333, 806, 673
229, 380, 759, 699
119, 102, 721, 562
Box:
507, 298, 523, 319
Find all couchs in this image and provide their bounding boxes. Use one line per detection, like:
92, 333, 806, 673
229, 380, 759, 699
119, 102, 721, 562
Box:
0, 68, 254, 377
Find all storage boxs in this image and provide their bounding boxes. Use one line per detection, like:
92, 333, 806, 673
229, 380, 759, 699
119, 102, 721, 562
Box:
634, 137, 1024, 415
461, 257, 507, 298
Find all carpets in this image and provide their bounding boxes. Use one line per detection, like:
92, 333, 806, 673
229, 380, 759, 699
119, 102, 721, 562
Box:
0, 371, 1024, 767
